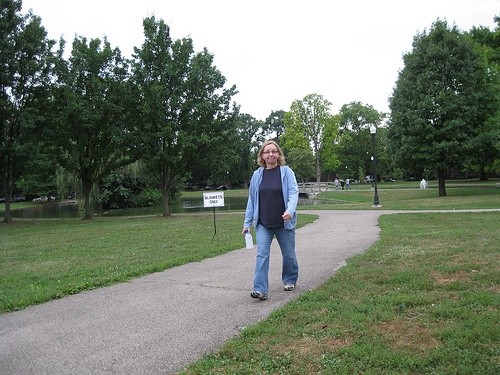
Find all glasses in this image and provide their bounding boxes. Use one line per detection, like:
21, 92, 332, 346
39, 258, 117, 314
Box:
262, 150, 278, 154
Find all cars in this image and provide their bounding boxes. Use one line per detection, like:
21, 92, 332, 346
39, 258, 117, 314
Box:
202, 186, 213, 190
32, 196, 56, 203
345, 179, 358, 184
364, 176, 375, 183
385, 178, 396, 182
217, 185, 227, 190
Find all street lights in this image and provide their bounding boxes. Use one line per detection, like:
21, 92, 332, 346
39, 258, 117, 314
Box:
226, 171, 229, 187
370, 123, 380, 206
371, 155, 374, 187
345, 165, 347, 180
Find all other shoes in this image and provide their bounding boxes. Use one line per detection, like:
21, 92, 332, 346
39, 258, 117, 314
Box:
284, 282, 296, 291
250, 291, 268, 300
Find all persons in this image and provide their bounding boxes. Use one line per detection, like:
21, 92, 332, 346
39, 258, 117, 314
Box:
242, 141, 299, 300
334, 177, 351, 191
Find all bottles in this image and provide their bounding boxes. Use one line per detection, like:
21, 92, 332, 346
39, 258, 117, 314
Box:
245, 231, 253, 249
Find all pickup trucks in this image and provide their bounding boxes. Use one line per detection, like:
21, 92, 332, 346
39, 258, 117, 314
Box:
0, 195, 25, 204
